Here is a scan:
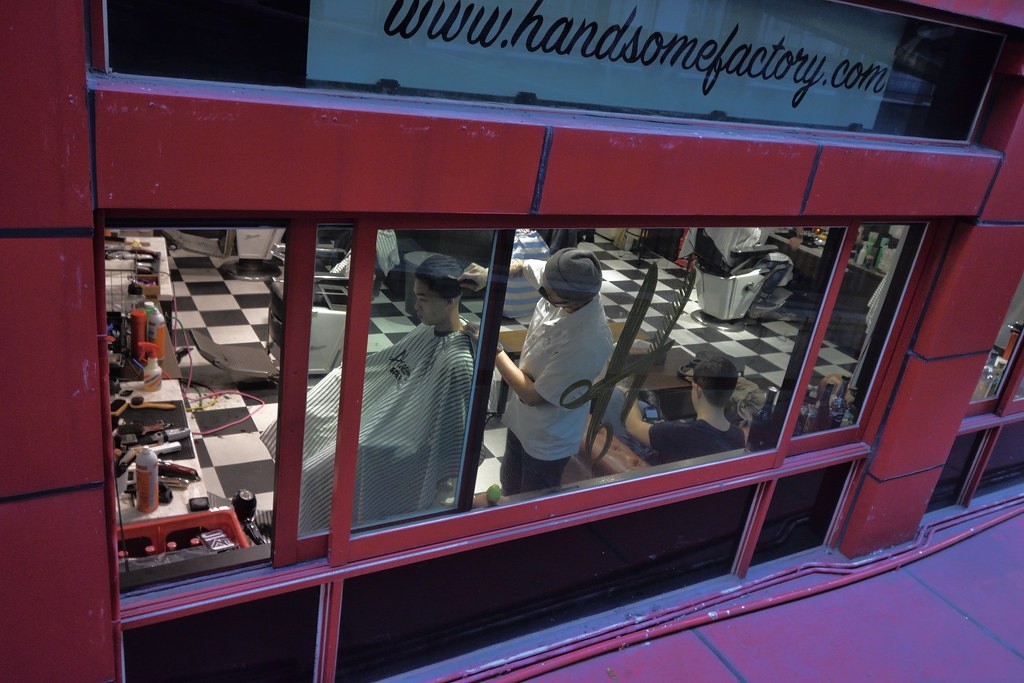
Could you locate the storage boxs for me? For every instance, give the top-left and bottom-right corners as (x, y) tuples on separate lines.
(116, 509), (250, 573)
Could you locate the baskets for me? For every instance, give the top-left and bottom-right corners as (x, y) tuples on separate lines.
(106, 249), (139, 320)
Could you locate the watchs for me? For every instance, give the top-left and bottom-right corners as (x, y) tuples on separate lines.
(497, 340), (504, 352)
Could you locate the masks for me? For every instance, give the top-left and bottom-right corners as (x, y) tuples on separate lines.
(538, 285), (573, 309)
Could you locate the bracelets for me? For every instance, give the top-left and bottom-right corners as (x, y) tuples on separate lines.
(624, 389), (629, 396)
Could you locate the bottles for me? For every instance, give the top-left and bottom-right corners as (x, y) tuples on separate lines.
(868, 231), (887, 269)
(124, 281), (145, 323)
(149, 308), (166, 360)
(855, 243), (868, 266)
(742, 374), (861, 456)
(136, 445), (159, 513)
(141, 302), (156, 317)
(124, 315), (133, 356)
(987, 361), (1007, 398)
(877, 236), (899, 274)
(969, 351), (1000, 403)
(138, 341), (162, 393)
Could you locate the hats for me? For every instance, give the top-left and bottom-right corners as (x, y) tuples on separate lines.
(544, 248), (602, 305)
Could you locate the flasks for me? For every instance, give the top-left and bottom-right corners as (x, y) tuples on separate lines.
(130, 310), (146, 357)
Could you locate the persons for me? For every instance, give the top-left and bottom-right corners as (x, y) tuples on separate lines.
(705, 227), (794, 311)
(590, 354), (747, 465)
(249, 252), (477, 546)
(456, 244), (615, 497)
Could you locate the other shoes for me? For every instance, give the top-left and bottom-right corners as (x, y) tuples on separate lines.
(755, 298), (778, 311)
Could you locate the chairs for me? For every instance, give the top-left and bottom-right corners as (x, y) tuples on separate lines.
(189, 235), (381, 380)
(693, 227), (794, 332)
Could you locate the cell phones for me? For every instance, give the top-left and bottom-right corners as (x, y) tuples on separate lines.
(644, 407), (661, 423)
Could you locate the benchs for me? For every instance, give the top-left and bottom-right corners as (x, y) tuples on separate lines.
(496, 320), (694, 422)
(497, 260), (696, 465)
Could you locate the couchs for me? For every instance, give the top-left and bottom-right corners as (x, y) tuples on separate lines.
(560, 412), (751, 483)
